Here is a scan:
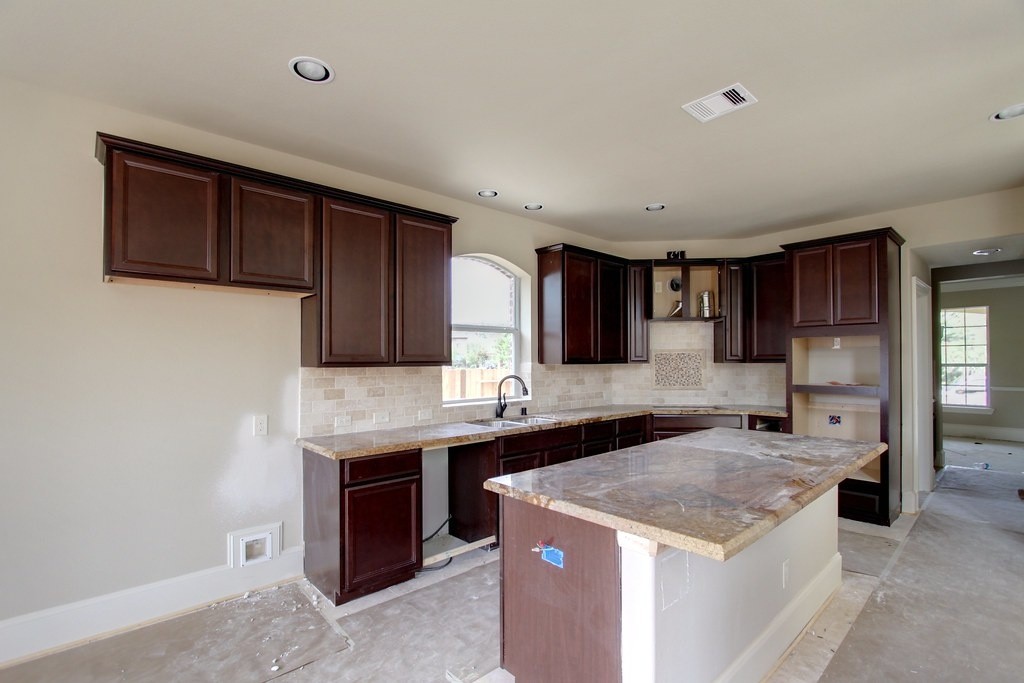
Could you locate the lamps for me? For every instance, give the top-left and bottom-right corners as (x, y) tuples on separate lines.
(644, 203), (666, 211)
(288, 56), (333, 85)
(477, 189), (499, 198)
(989, 103), (1024, 121)
(523, 203), (543, 211)
(973, 248), (1002, 256)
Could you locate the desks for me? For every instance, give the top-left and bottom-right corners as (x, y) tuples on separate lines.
(482, 425), (888, 683)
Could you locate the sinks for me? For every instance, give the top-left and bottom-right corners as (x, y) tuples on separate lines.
(509, 416), (557, 426)
(472, 420), (527, 432)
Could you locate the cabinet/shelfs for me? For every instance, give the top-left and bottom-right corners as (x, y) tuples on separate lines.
(714, 250), (788, 363)
(747, 415), (789, 434)
(101, 150), (315, 299)
(302, 448), (423, 606)
(649, 414), (747, 442)
(647, 260), (725, 321)
(299, 194), (452, 369)
(448, 413), (649, 552)
(535, 242), (649, 364)
(791, 238), (901, 526)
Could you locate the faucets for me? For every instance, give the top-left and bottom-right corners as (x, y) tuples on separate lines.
(495, 375), (528, 418)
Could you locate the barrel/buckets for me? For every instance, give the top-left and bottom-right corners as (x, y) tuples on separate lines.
(697, 290), (715, 318)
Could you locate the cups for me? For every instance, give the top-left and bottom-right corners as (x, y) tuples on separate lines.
(669, 300), (682, 317)
